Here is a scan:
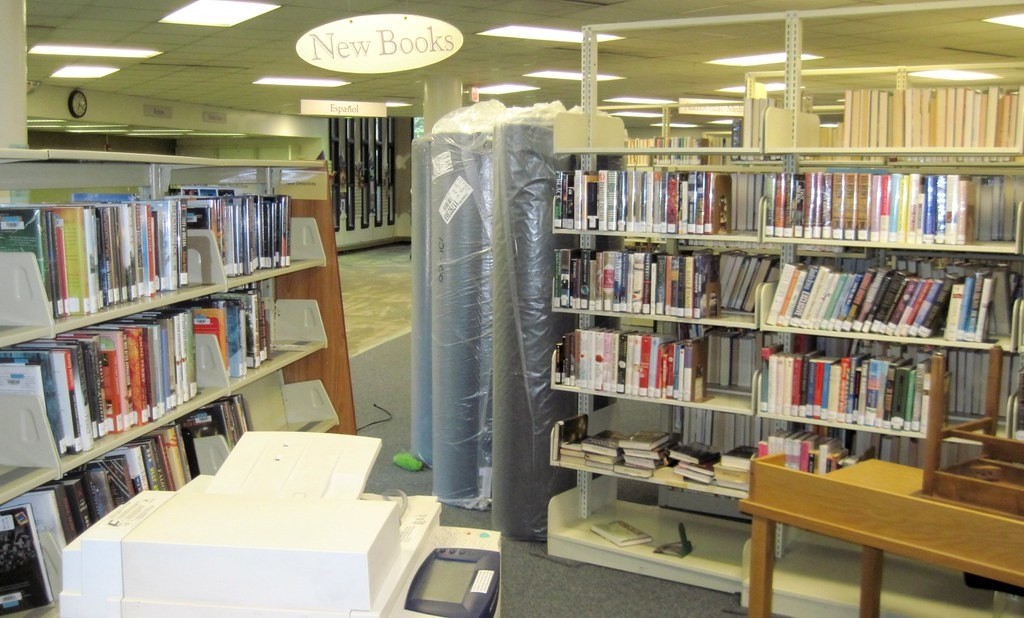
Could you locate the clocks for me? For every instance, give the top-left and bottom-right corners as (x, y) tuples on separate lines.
(68, 90), (87, 118)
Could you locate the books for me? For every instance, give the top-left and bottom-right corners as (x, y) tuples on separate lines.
(0, 194), (292, 615)
(590, 520), (652, 548)
(553, 88), (1024, 492)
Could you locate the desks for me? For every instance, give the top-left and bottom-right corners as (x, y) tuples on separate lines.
(739, 452), (1024, 618)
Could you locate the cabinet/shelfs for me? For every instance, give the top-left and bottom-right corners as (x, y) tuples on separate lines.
(0, 150), (358, 618)
(547, 0), (1024, 618)
